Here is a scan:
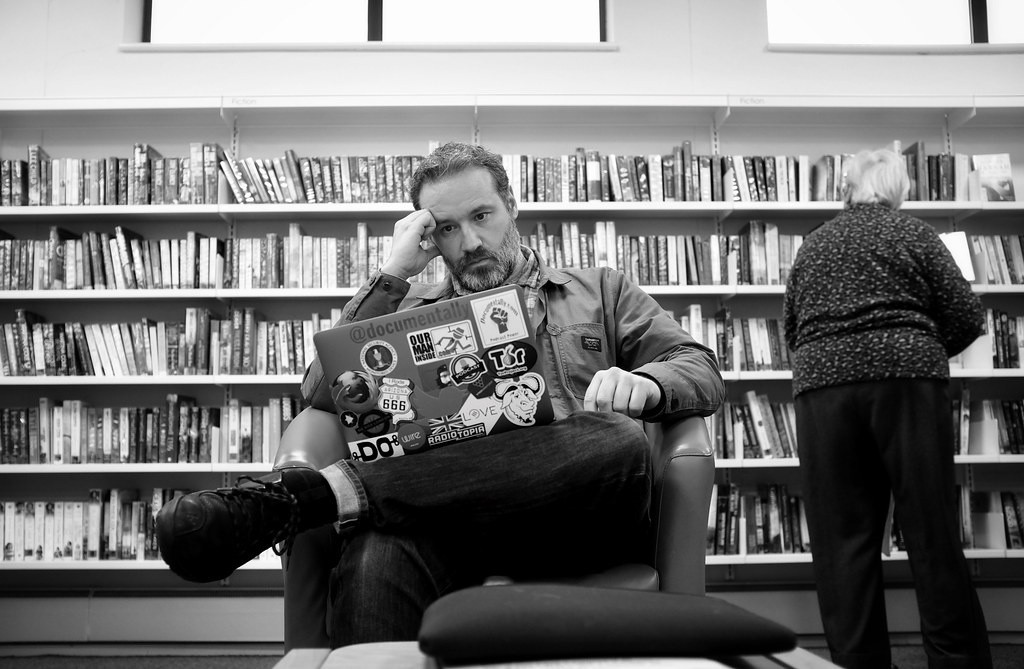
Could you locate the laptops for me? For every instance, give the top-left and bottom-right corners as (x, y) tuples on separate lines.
(313, 284), (556, 460)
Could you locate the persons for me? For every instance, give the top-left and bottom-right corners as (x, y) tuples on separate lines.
(155, 138), (726, 649)
(781, 146), (1003, 669)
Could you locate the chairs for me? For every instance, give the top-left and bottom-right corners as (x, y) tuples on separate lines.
(274, 405), (713, 653)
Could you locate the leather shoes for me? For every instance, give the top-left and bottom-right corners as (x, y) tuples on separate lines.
(156, 467), (339, 583)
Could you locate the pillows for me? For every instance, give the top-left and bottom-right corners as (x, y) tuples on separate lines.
(415, 586), (795, 661)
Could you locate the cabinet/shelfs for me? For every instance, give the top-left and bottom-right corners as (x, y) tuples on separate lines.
(1, 200), (1023, 596)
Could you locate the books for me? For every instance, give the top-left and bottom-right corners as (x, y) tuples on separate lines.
(0, 144), (1024, 566)
